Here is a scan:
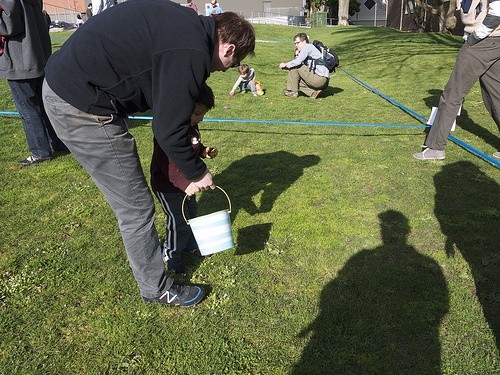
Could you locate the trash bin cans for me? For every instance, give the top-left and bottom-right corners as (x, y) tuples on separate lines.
(311, 13), (316, 28)
(315, 12), (327, 28)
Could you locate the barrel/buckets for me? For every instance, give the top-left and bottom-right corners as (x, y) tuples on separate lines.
(182, 185), (235, 256)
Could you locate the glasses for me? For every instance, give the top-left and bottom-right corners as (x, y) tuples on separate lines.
(231, 49), (240, 68)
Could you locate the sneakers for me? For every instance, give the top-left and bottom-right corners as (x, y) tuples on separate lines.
(141, 283), (205, 308)
(493, 151), (500, 159)
(412, 145), (446, 160)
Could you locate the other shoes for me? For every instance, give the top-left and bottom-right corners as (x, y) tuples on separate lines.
(18, 155), (53, 166)
(309, 89), (322, 98)
(183, 247), (201, 257)
(169, 265), (187, 277)
(284, 89), (299, 97)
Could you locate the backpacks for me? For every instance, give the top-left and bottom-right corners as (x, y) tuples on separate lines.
(306, 39), (339, 74)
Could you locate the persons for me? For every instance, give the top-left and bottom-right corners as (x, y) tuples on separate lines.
(0, 0), (69, 166)
(279, 33), (330, 99)
(149, 82), (218, 271)
(230, 63), (265, 98)
(76, 0), (120, 29)
(41, 10), (51, 32)
(42, 0), (255, 309)
(178, 0), (224, 16)
(412, 0), (500, 161)
